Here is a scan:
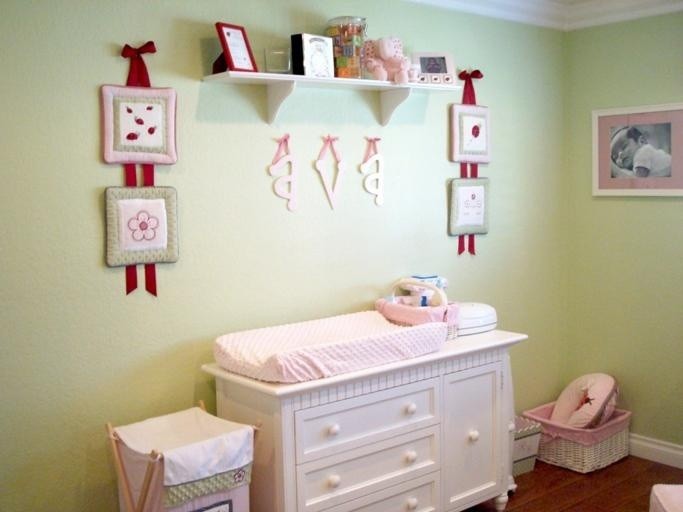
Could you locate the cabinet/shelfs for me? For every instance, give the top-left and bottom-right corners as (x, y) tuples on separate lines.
(199, 333), (527, 511)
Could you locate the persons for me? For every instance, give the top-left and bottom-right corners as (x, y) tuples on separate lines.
(610, 127), (671, 177)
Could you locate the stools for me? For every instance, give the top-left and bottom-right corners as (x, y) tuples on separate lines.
(647, 484), (682, 512)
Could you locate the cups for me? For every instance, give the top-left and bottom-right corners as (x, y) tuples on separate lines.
(264, 47), (291, 73)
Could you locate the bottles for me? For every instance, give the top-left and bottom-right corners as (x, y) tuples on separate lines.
(325, 15), (368, 78)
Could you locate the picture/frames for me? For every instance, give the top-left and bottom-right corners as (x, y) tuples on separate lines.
(211, 18), (257, 71)
(591, 101), (683, 196)
(412, 50), (457, 85)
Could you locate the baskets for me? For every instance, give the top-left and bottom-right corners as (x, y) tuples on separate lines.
(523, 401), (631, 474)
(377, 280), (447, 326)
